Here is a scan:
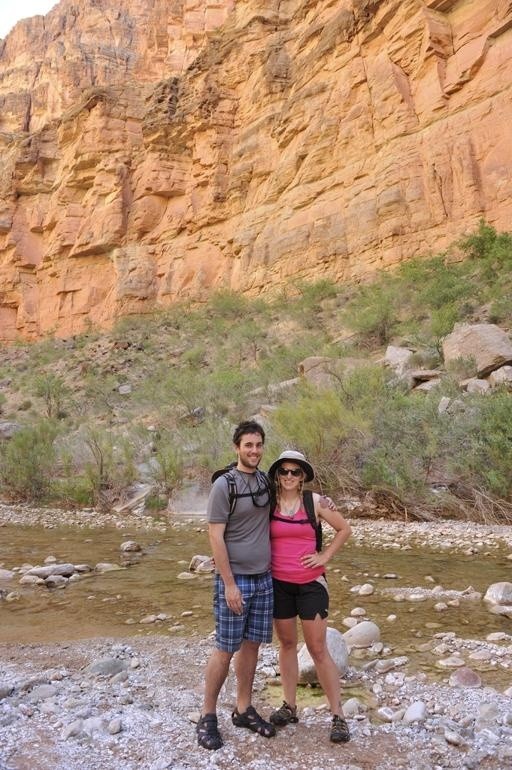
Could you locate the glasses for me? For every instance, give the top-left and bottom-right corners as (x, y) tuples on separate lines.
(276, 467), (303, 477)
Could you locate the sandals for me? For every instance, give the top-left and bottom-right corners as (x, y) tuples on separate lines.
(269, 700), (299, 726)
(231, 705), (276, 738)
(195, 712), (224, 750)
(330, 714), (351, 743)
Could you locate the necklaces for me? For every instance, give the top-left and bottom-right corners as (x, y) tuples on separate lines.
(281, 496), (299, 518)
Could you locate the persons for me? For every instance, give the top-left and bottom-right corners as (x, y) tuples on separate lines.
(195, 420), (337, 749)
(210, 450), (351, 741)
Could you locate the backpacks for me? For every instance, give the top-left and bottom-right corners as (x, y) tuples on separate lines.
(270, 490), (322, 555)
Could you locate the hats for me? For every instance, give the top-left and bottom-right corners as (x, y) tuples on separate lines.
(211, 461), (239, 485)
(267, 449), (315, 484)
